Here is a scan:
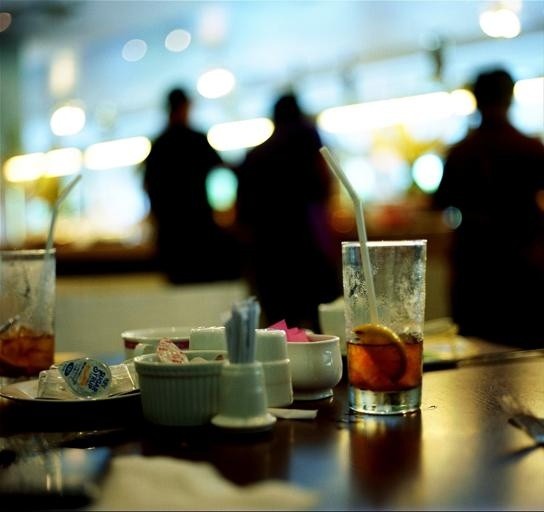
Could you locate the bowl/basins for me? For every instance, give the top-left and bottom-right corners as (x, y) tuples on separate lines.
(134, 350), (229, 427)
(120, 326), (198, 360)
(189, 328), (294, 408)
(286, 334), (344, 401)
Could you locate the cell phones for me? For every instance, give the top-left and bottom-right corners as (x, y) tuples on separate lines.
(0, 445), (111, 506)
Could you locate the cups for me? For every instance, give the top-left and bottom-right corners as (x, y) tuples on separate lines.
(1, 247), (57, 379)
(341, 239), (428, 416)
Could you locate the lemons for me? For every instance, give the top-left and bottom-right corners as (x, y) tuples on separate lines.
(353, 323), (408, 383)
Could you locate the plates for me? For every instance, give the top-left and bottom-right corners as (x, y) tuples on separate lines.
(1, 362), (142, 401)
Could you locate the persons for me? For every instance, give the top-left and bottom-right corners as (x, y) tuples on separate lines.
(427, 68), (543, 352)
